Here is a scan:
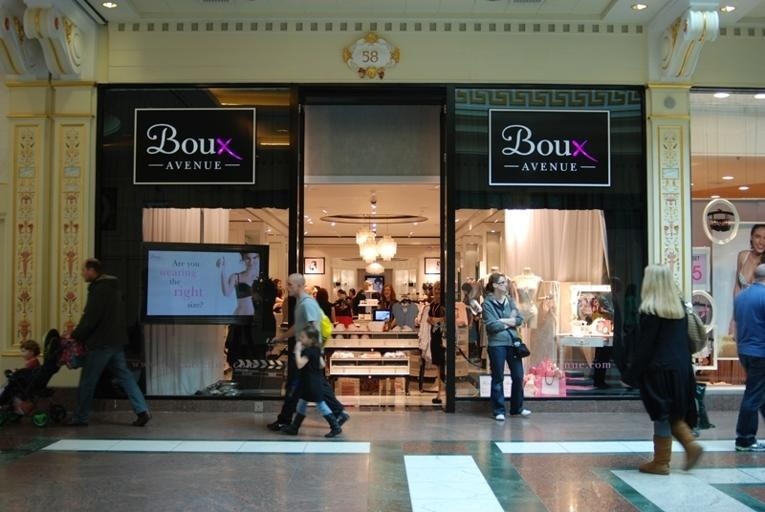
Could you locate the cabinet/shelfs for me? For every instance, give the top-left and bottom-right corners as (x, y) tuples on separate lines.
(324, 285), (426, 395)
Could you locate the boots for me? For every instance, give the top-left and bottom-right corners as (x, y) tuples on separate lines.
(672, 421), (702, 470)
(638, 437), (672, 475)
(323, 414), (342, 437)
(278, 413), (306, 435)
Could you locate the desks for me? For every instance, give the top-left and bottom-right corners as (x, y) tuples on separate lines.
(556, 332), (613, 371)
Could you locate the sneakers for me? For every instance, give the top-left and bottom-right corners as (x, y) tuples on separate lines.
(132, 409), (151, 426)
(521, 409), (532, 417)
(736, 440), (765, 452)
(495, 413), (505, 420)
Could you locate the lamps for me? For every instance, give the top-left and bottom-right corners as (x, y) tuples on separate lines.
(355, 216), (397, 274)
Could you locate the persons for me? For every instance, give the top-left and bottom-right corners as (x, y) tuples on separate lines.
(215, 252), (259, 316)
(64, 258), (152, 428)
(272, 273), (482, 438)
(732, 263), (765, 452)
(482, 272), (532, 420)
(728, 224), (765, 337)
(19, 340), (41, 368)
(638, 263), (704, 476)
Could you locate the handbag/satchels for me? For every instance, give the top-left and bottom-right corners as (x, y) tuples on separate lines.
(535, 376), (567, 398)
(320, 314), (334, 349)
(686, 301), (706, 354)
(511, 337), (530, 359)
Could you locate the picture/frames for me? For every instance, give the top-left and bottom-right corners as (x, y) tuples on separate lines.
(691, 326), (717, 371)
(712, 221), (765, 361)
(424, 257), (440, 274)
(692, 247), (711, 293)
(303, 256), (325, 275)
(139, 241), (270, 324)
(693, 290), (716, 334)
(570, 285), (614, 330)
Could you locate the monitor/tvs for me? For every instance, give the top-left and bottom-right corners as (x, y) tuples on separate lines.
(374, 309), (391, 320)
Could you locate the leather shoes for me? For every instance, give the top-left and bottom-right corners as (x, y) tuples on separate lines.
(336, 412), (349, 427)
(267, 420), (291, 431)
